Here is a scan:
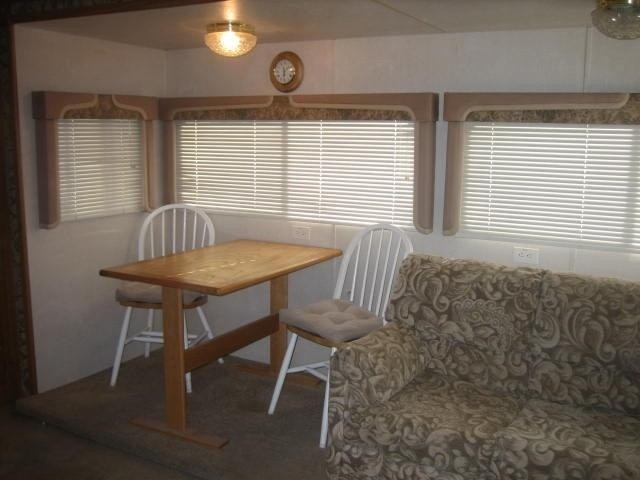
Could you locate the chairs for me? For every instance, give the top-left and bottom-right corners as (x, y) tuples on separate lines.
(109, 203), (226, 395)
(266, 222), (416, 451)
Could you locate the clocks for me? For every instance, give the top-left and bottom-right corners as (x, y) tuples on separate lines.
(268, 51), (304, 92)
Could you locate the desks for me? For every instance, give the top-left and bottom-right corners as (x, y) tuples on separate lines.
(100, 240), (345, 451)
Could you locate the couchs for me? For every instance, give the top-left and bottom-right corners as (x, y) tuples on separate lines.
(320, 252), (639, 478)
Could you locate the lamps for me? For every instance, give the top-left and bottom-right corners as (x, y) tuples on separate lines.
(590, 1), (639, 41)
(203, 22), (257, 59)
